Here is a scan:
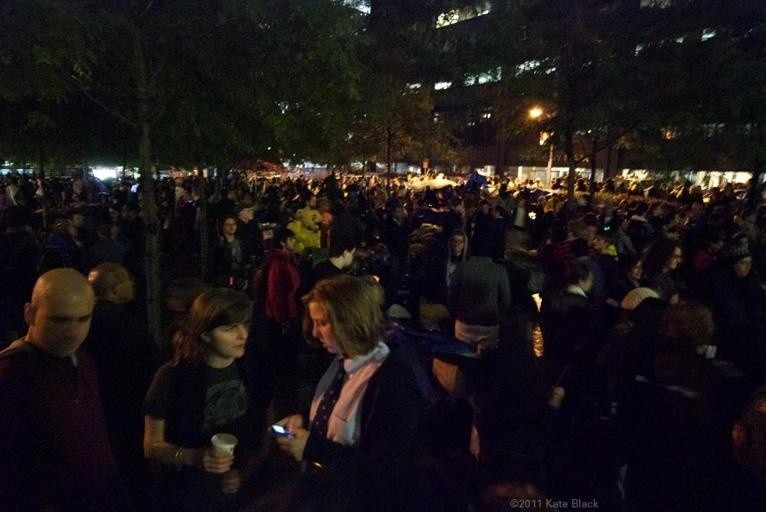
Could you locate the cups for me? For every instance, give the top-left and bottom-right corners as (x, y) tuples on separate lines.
(211, 434), (237, 456)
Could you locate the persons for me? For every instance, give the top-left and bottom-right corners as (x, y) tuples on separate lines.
(0, 162), (766, 512)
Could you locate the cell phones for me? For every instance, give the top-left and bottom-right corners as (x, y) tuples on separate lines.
(271, 424), (293, 440)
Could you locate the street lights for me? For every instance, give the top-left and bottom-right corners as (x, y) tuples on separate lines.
(528, 106), (554, 184)
